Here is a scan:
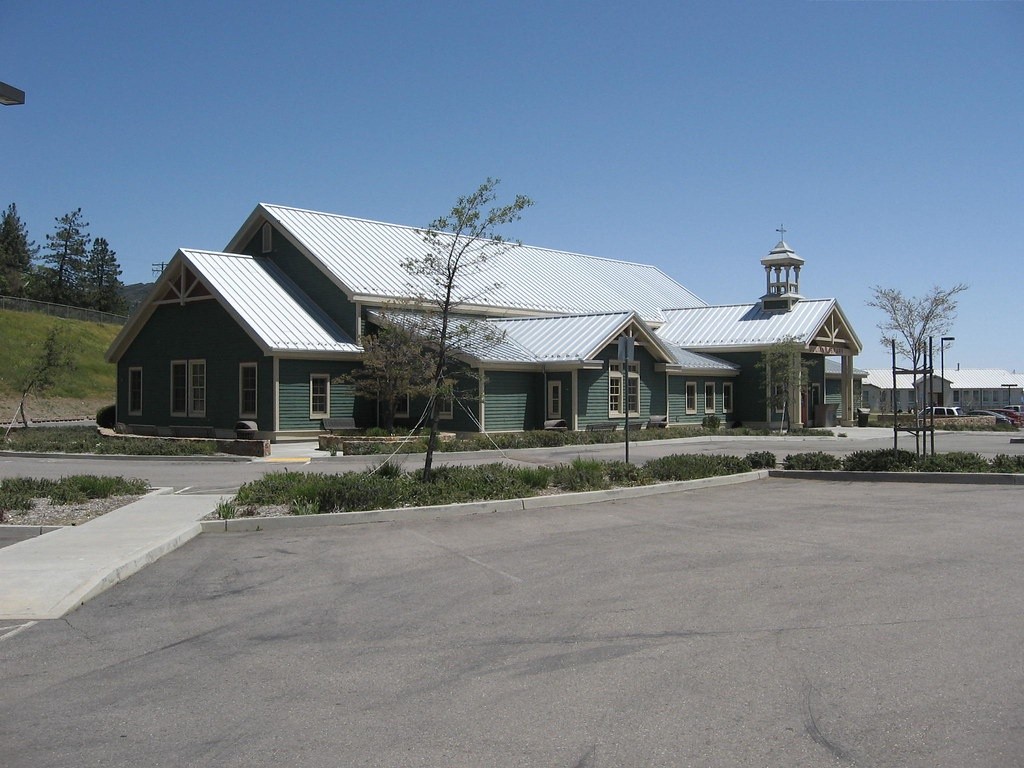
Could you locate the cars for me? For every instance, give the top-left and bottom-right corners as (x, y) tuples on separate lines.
(965, 405), (1024, 429)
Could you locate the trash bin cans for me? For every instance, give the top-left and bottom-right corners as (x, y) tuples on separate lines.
(650, 416), (667, 428)
(233, 421), (259, 440)
(544, 419), (567, 432)
(857, 408), (870, 427)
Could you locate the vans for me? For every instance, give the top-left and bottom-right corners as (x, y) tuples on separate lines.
(918, 406), (965, 417)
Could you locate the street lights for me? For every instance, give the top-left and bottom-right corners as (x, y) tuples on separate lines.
(1001, 383), (1018, 405)
(941, 335), (956, 406)
